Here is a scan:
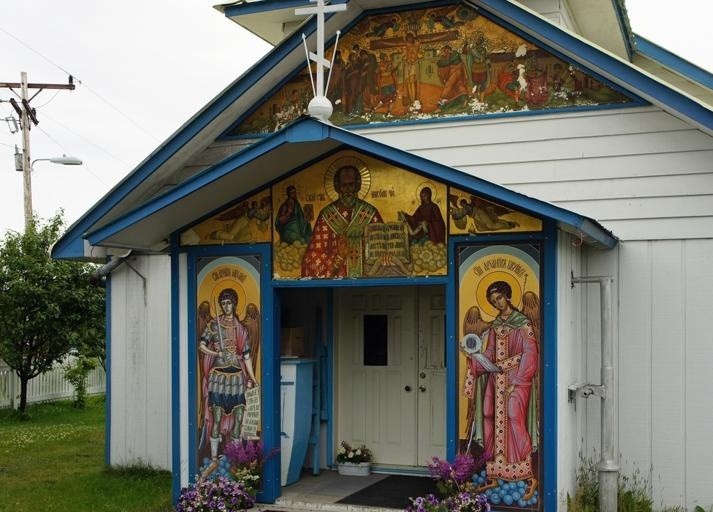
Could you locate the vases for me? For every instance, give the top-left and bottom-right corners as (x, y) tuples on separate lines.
(337, 462), (370, 475)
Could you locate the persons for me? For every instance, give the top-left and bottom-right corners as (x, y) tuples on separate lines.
(194, 288), (257, 465)
(299, 165), (386, 280)
(397, 186), (445, 248)
(447, 199), (520, 231)
(287, 90), (305, 115)
(454, 278), (538, 501)
(203, 201), (271, 242)
(272, 185), (313, 244)
(320, 13), (568, 112)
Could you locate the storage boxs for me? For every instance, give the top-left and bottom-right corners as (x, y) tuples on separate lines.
(280, 326), (305, 357)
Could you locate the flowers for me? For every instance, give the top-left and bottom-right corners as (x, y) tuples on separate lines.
(335, 439), (373, 463)
(404, 452), (494, 512)
(177, 437), (279, 512)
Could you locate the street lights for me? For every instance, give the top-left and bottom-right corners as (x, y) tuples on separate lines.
(21, 72), (82, 235)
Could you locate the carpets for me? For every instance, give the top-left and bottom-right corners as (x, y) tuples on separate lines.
(334, 475), (448, 508)
